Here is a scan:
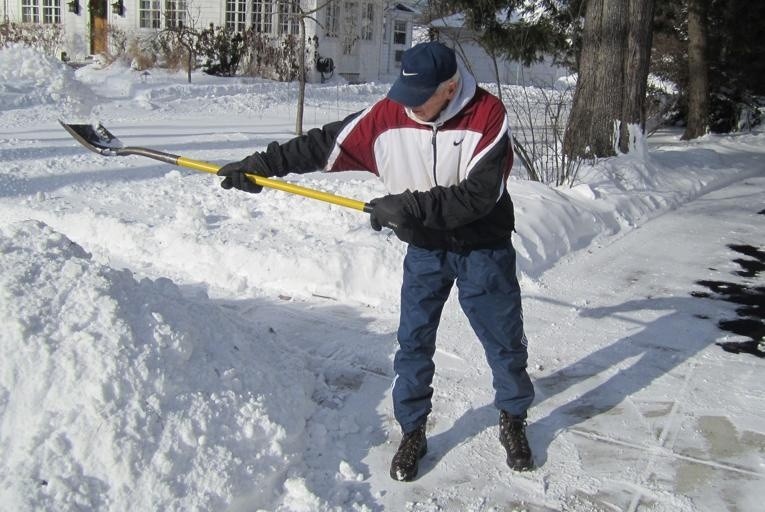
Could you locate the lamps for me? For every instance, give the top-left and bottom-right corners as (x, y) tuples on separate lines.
(111, 0), (123, 15)
(65, 0), (80, 14)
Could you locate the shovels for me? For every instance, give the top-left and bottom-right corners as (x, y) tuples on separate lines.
(57, 118), (377, 215)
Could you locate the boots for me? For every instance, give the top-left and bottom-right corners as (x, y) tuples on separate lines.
(388, 411), (428, 484)
(499, 408), (533, 472)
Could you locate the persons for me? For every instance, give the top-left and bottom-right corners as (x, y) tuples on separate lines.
(216, 41), (536, 482)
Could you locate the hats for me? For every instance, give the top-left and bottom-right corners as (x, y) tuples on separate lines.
(386, 41), (459, 107)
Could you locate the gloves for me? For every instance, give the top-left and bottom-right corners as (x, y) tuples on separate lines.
(216, 151), (272, 194)
(366, 188), (423, 233)
